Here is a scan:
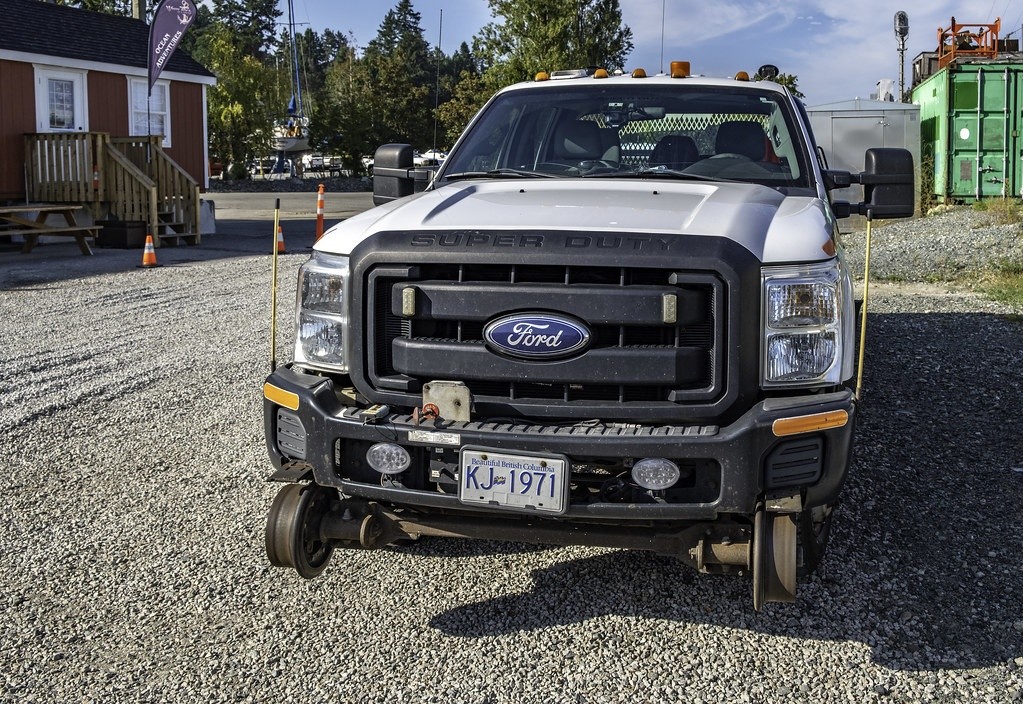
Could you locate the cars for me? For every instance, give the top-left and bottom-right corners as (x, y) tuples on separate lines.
(225, 150), (374, 181)
(412, 148), (448, 168)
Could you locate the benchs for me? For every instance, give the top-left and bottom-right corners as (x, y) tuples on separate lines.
(0, 225), (104, 237)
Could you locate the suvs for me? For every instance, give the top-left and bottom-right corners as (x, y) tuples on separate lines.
(260, 59), (915, 612)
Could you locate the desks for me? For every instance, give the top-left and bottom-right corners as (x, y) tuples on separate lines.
(0, 204), (93, 256)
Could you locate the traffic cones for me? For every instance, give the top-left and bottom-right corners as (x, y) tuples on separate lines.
(136, 236), (164, 268)
(269, 226), (288, 255)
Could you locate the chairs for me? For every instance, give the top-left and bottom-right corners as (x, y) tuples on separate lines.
(596, 127), (621, 164)
(648, 135), (698, 171)
(556, 120), (601, 168)
(713, 120), (769, 164)
